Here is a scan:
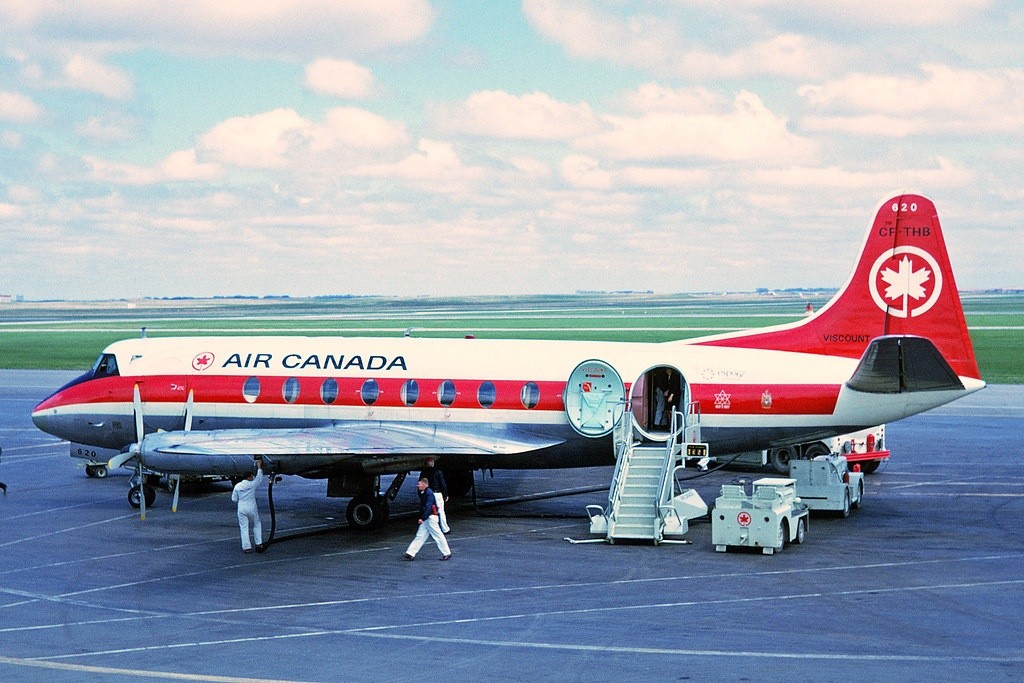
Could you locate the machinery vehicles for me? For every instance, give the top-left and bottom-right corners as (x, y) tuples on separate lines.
(711, 478), (809, 554)
(789, 451), (865, 518)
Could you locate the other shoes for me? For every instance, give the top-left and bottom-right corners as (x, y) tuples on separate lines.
(441, 553), (453, 560)
(255, 545), (264, 553)
(243, 548), (253, 554)
(403, 552), (414, 560)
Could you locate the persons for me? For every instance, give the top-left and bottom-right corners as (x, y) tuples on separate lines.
(663, 368), (680, 426)
(654, 369), (666, 427)
(418, 458), (452, 535)
(402, 478), (452, 561)
(0, 447), (7, 494)
(232, 461), (265, 553)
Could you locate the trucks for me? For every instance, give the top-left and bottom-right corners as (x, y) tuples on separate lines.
(704, 425), (890, 475)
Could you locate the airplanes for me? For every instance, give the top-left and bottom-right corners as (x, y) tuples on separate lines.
(31, 195), (987, 531)
